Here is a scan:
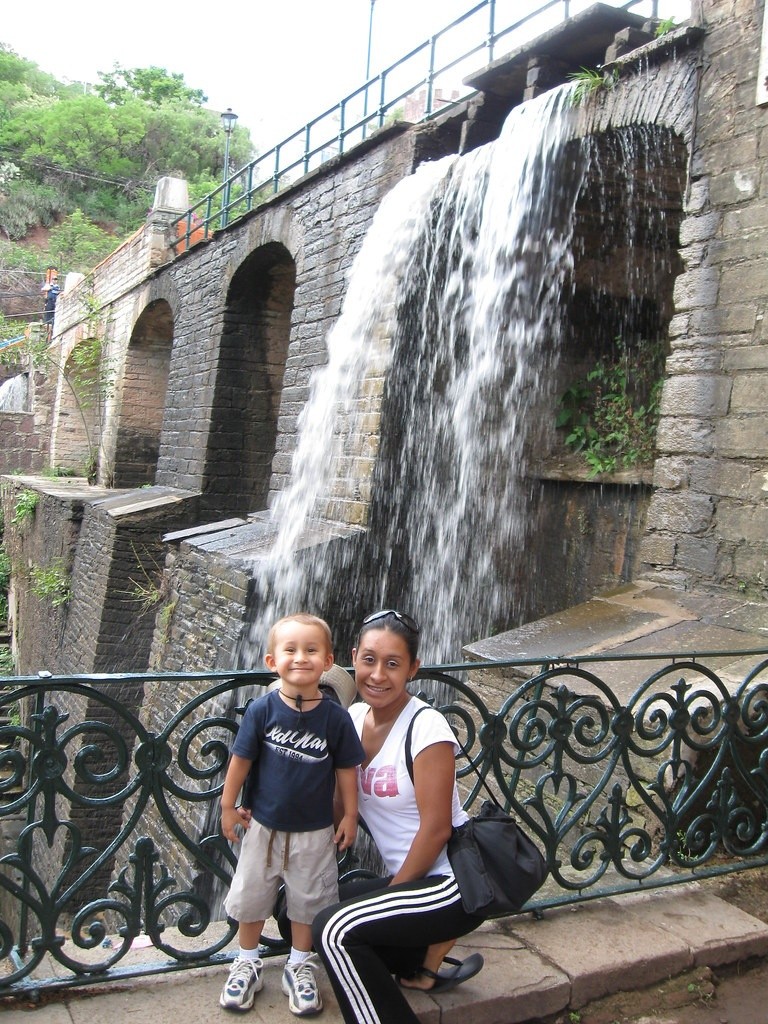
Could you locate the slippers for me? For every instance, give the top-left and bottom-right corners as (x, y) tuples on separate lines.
(395, 952), (484, 994)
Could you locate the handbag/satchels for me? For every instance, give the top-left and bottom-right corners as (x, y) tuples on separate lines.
(404, 706), (549, 915)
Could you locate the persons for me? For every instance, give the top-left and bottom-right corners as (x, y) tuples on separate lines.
(219, 614), (367, 1016)
(237, 610), (484, 1024)
(45, 276), (61, 324)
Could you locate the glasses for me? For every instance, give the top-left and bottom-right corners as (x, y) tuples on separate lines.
(362, 609), (420, 636)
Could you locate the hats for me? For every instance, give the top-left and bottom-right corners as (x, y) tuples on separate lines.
(264, 664), (358, 711)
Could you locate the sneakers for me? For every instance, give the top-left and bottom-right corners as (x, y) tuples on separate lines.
(219, 957), (264, 1010)
(282, 952), (324, 1015)
